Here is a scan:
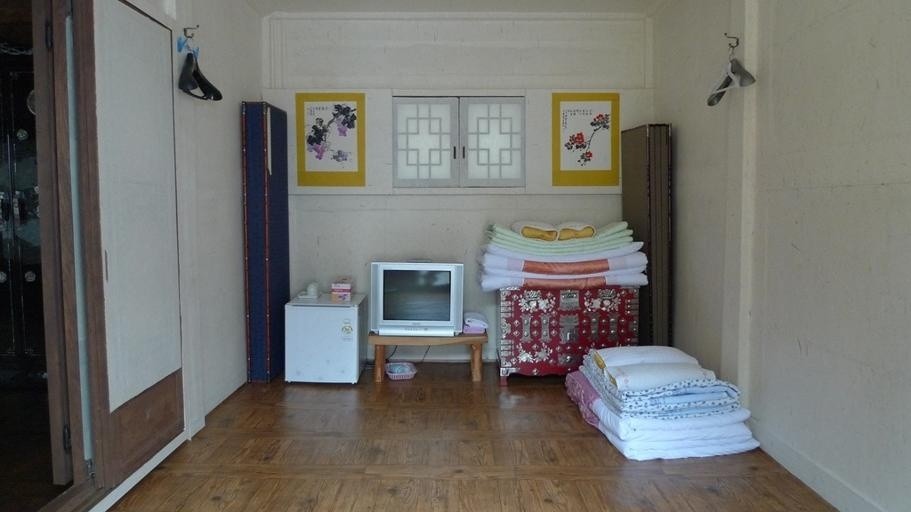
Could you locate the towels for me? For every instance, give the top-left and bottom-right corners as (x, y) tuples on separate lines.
(464, 311), (487, 333)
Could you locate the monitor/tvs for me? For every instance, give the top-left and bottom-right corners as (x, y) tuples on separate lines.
(368, 262), (463, 337)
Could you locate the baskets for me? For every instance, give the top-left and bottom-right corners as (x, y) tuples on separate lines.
(385, 362), (416, 380)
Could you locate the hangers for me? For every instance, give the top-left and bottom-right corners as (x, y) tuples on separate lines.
(704, 42), (758, 108)
(179, 35), (223, 102)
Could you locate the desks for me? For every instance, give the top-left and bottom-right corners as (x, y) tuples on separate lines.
(368, 329), (487, 383)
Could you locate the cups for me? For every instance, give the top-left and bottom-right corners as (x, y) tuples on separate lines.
(307, 281), (321, 296)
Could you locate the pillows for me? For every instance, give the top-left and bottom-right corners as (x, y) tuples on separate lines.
(512, 221), (599, 241)
(594, 344), (717, 392)
